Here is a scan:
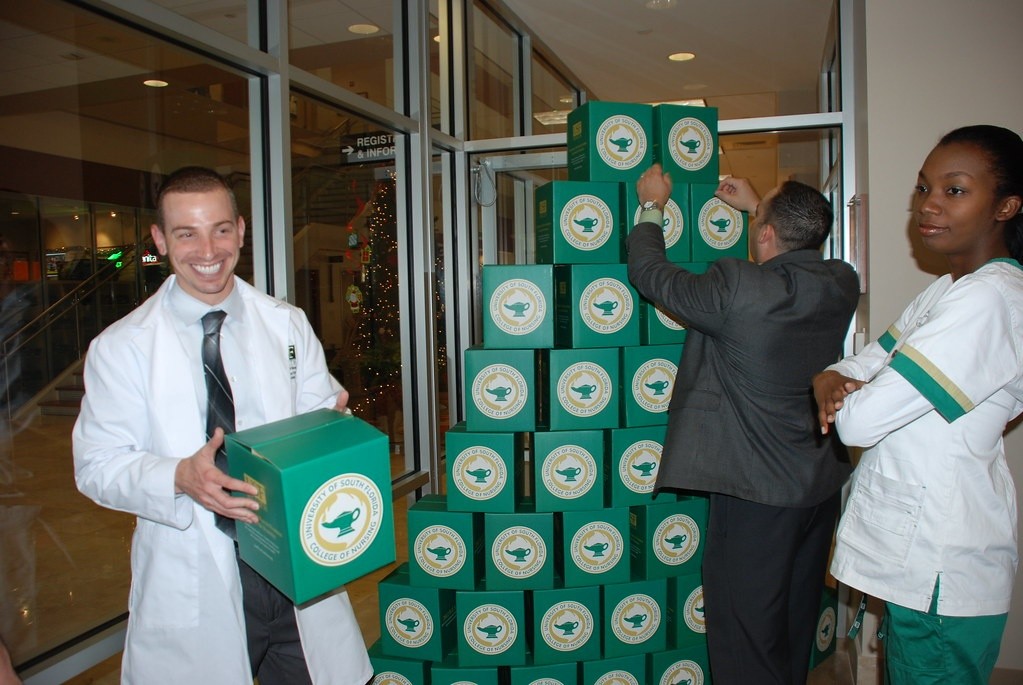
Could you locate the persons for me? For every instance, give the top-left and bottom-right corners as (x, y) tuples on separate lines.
(811, 125), (1023, 685)
(69, 165), (379, 685)
(626, 164), (859, 685)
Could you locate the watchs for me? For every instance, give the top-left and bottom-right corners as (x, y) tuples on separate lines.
(641, 200), (664, 215)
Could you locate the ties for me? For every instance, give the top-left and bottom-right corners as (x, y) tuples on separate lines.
(199, 310), (238, 542)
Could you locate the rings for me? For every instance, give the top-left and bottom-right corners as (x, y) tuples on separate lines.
(640, 176), (644, 178)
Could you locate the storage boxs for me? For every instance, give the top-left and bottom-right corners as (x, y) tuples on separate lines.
(224, 100), (837, 685)
